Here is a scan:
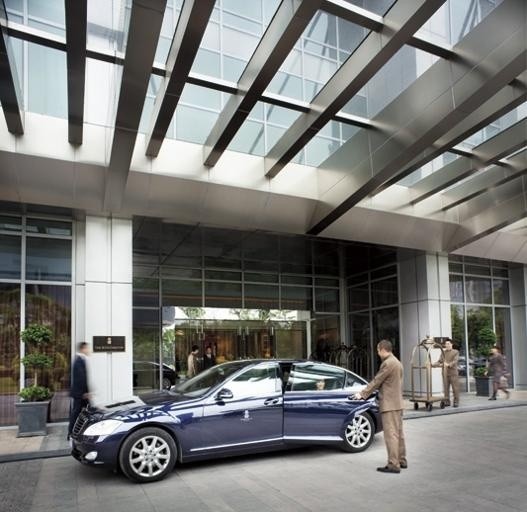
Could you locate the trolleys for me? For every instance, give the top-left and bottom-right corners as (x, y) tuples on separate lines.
(328, 343), (369, 383)
(407, 336), (450, 412)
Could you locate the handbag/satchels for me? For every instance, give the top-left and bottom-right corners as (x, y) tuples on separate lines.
(499, 375), (509, 388)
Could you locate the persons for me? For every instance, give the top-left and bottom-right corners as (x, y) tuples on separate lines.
(283, 369), (291, 390)
(204, 346), (216, 371)
(316, 379), (325, 389)
(434, 339), (461, 408)
(352, 340), (407, 473)
(315, 331), (330, 360)
(187, 345), (199, 379)
(65, 341), (93, 442)
(484, 346), (510, 400)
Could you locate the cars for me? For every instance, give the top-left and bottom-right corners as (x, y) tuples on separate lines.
(456, 357), (475, 375)
(132, 361), (178, 392)
(68, 356), (384, 484)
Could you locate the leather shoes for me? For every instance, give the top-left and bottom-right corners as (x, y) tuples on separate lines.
(377, 465), (401, 473)
(443, 391), (511, 407)
(399, 463), (408, 468)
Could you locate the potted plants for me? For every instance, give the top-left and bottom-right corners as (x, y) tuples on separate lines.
(14, 324), (57, 436)
(473, 326), (497, 398)
